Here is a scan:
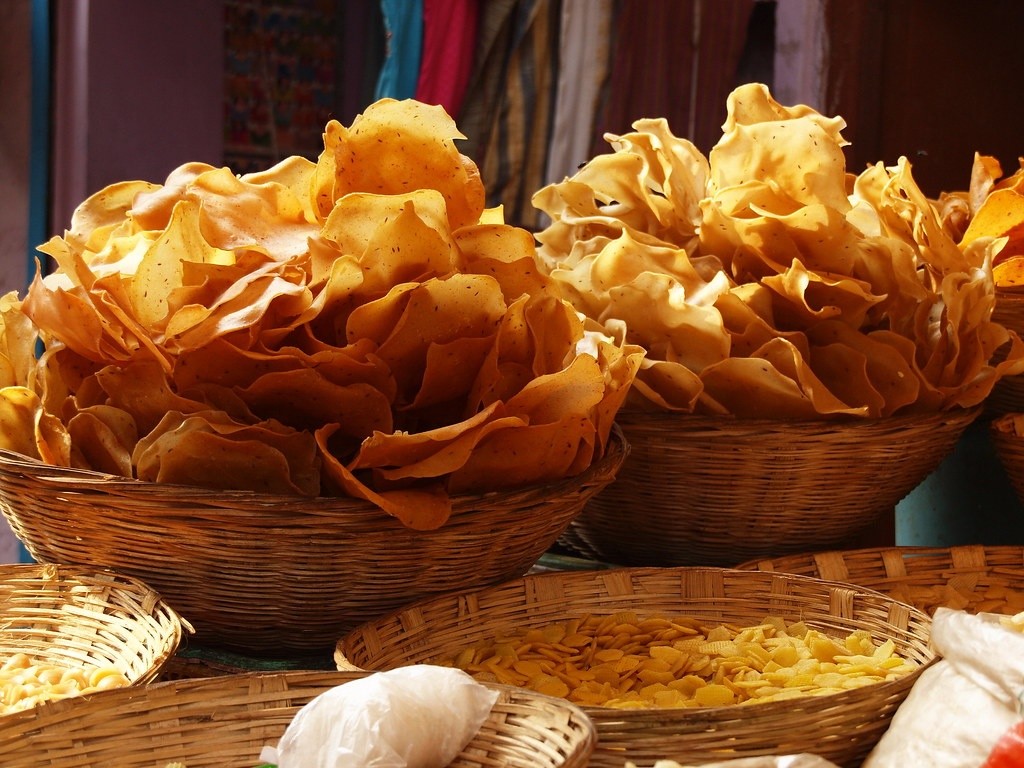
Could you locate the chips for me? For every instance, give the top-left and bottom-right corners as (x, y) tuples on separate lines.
(1, 83), (1024, 533)
(434, 613), (917, 710)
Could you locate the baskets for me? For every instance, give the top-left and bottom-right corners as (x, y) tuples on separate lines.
(1, 408), (1024, 768)
